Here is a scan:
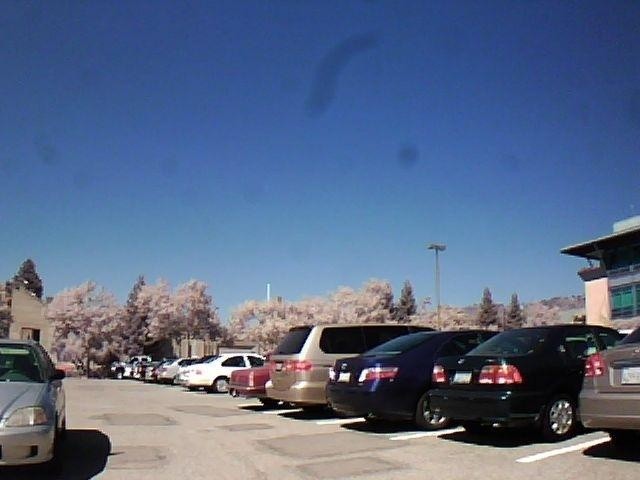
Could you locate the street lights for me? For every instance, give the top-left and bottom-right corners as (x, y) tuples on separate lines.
(428, 243), (445, 330)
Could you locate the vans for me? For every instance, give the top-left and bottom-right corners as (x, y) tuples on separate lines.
(262, 320), (439, 416)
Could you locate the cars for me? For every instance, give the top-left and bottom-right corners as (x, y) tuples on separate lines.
(111, 352), (269, 399)
(579, 324), (640, 451)
(326, 327), (501, 429)
(0, 339), (67, 471)
(428, 323), (627, 437)
